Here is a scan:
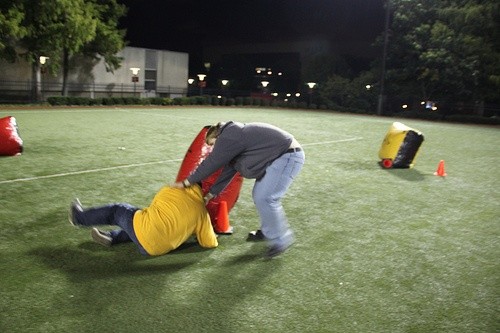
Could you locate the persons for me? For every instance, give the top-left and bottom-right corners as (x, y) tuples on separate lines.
(70, 179), (219, 257)
(183, 121), (305, 256)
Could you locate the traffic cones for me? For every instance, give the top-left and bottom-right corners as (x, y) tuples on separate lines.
(433, 160), (448, 177)
(213, 200), (234, 235)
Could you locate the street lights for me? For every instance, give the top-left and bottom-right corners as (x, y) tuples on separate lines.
(221, 79), (229, 97)
(261, 81), (270, 96)
(130, 67), (141, 97)
(197, 73), (207, 96)
(306, 82), (317, 108)
(188, 78), (195, 97)
(39, 55), (49, 102)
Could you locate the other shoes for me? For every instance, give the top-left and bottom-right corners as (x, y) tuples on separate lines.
(91, 228), (113, 246)
(249, 229), (265, 240)
(69, 198), (84, 228)
(268, 237), (295, 257)
(214, 227), (233, 235)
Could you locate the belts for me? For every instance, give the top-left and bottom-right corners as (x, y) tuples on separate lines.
(285, 148), (301, 153)
